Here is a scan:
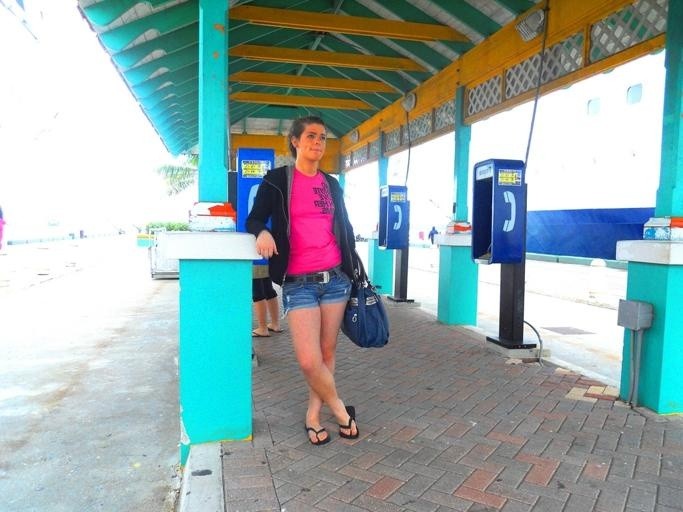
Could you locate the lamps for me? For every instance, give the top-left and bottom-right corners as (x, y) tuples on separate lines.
(514, 9), (546, 41)
(400, 92), (416, 113)
(349, 130), (359, 144)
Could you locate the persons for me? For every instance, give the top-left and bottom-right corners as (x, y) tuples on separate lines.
(252, 279), (284, 338)
(429, 226), (438, 244)
(246, 116), (359, 446)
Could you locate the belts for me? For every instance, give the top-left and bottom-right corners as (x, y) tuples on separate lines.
(285, 268), (343, 284)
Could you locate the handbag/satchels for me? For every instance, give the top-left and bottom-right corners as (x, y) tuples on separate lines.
(341, 280), (389, 348)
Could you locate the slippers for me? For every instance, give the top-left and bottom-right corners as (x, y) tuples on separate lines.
(305, 424), (330, 445)
(252, 331), (270, 337)
(340, 405), (359, 439)
(267, 327), (283, 333)
